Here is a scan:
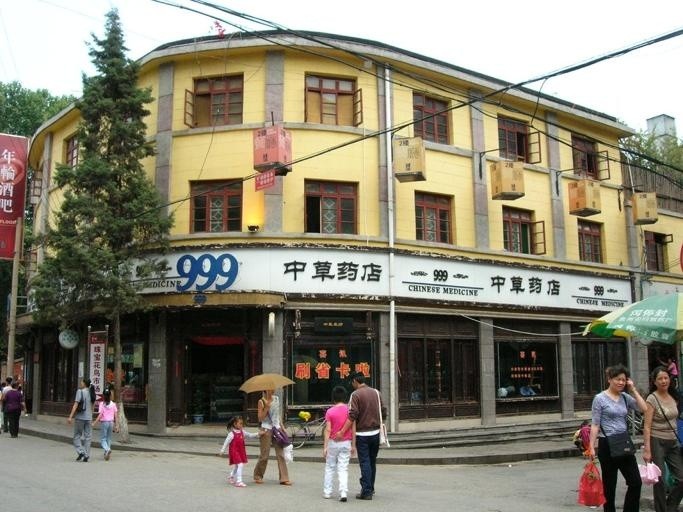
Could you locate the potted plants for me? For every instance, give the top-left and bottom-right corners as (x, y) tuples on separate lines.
(190, 386), (204, 425)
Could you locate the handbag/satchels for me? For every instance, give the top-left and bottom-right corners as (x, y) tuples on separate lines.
(379, 424), (390, 448)
(75, 397), (86, 412)
(607, 431), (636, 457)
(272, 426), (290, 448)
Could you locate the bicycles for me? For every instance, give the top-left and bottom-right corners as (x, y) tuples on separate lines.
(284, 403), (329, 451)
(626, 407), (644, 437)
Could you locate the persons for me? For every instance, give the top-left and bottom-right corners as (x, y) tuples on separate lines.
(585, 363), (648, 512)
(67, 377), (95, 461)
(218, 415), (266, 488)
(642, 365), (682, 512)
(0, 376), (28, 441)
(335, 369), (387, 500)
(92, 388), (118, 461)
(252, 391), (293, 485)
(656, 357), (678, 388)
(321, 386), (357, 501)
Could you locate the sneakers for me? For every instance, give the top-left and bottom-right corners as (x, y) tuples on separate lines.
(356, 494), (373, 500)
(281, 481), (292, 485)
(338, 497), (347, 501)
(104, 448), (112, 460)
(76, 452), (89, 462)
(255, 479), (263, 483)
(227, 476), (247, 487)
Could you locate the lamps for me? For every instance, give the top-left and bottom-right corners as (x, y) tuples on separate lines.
(246, 222), (261, 234)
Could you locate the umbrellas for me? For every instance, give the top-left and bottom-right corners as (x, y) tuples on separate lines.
(580, 289), (683, 404)
(237, 372), (295, 400)
(636, 459), (662, 487)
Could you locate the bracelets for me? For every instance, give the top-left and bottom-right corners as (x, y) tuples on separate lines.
(628, 387), (636, 393)
